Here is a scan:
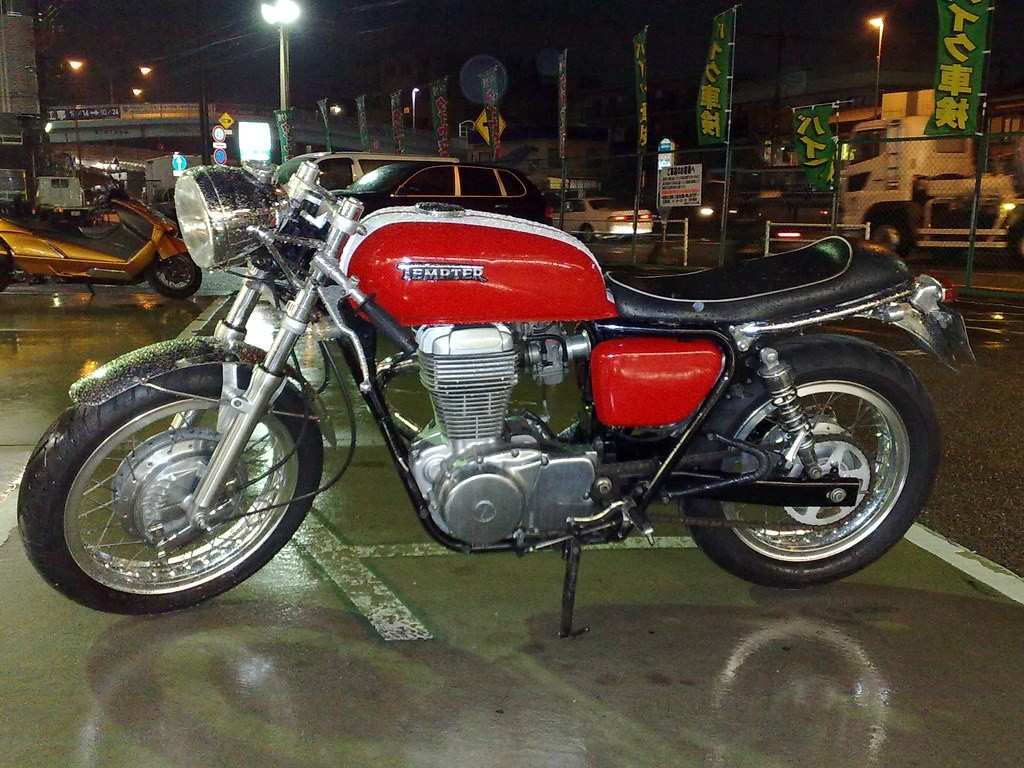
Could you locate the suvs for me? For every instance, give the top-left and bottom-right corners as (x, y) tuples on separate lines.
(277, 145), (458, 193)
(326, 158), (555, 230)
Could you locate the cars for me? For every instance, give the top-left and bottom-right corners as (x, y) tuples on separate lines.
(549, 195), (656, 244)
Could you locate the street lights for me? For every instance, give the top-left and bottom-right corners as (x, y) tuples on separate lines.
(869, 15), (887, 156)
(260, 0), (304, 162)
(140, 63), (163, 119)
(64, 56), (88, 159)
(412, 86), (422, 155)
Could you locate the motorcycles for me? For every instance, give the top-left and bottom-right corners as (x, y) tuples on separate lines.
(0, 160), (204, 300)
(14, 158), (977, 641)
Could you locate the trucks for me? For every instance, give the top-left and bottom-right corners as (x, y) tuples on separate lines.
(838, 113), (1024, 276)
